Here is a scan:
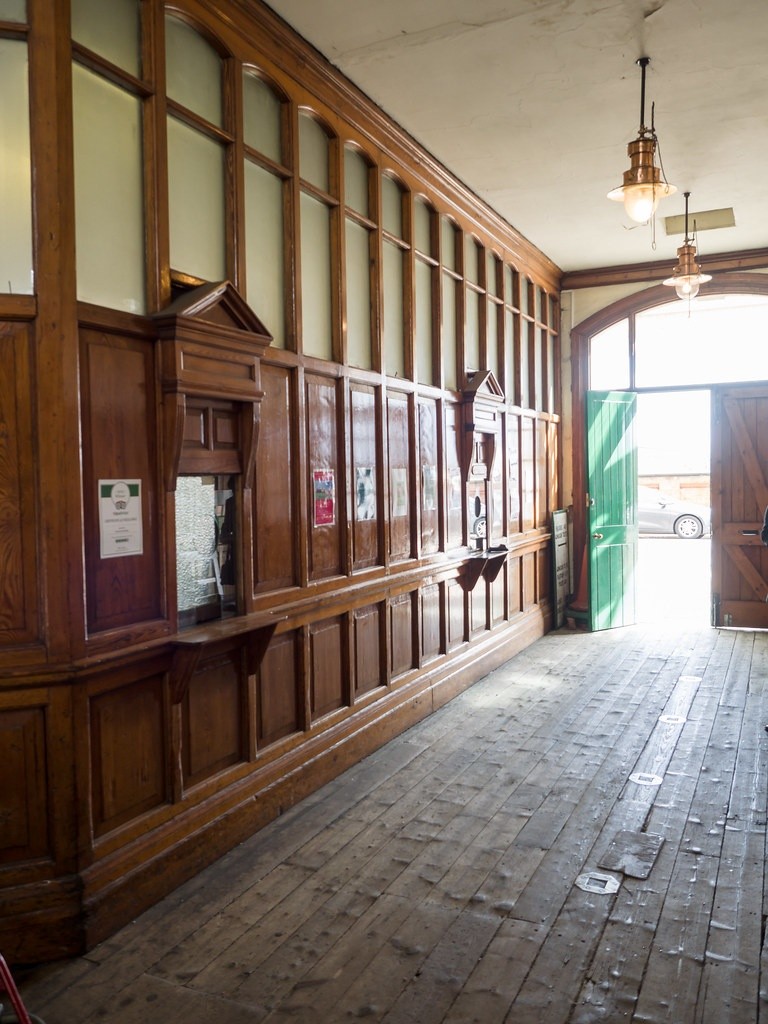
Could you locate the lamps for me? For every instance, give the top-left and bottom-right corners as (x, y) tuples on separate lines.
(608, 52), (678, 224)
(662, 192), (713, 302)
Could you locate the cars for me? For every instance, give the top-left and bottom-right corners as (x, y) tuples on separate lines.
(636, 485), (711, 540)
